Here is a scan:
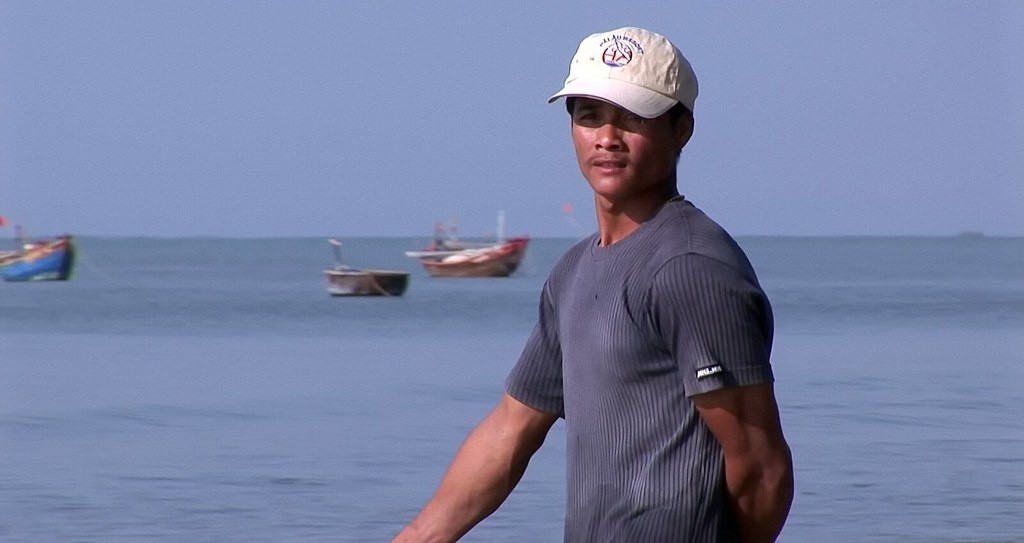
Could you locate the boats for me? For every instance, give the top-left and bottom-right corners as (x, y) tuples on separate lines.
(403, 209), (531, 278)
(320, 238), (412, 298)
(0, 215), (75, 282)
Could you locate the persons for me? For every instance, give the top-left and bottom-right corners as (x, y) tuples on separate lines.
(388, 28), (796, 543)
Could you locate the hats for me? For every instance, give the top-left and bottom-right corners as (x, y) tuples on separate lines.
(547, 26), (699, 120)
